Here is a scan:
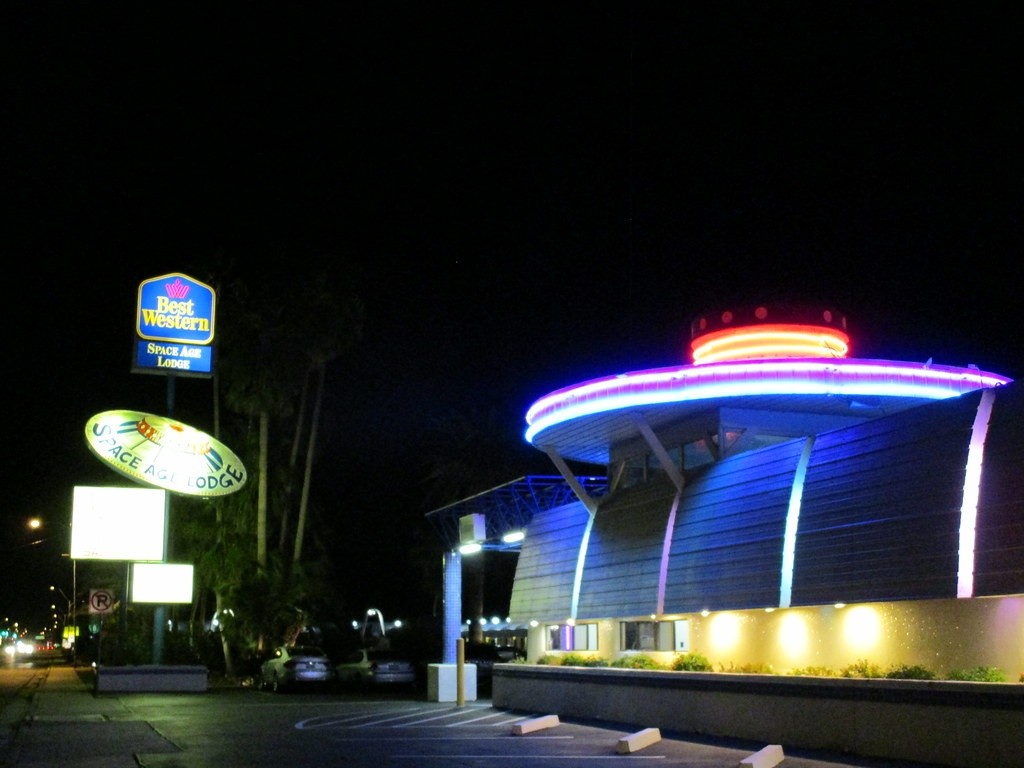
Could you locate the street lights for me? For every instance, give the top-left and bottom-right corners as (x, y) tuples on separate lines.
(49, 586), (71, 633)
(29, 518), (78, 667)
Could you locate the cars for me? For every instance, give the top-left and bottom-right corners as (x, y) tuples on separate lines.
(464, 644), (525, 684)
(255, 644), (416, 696)
